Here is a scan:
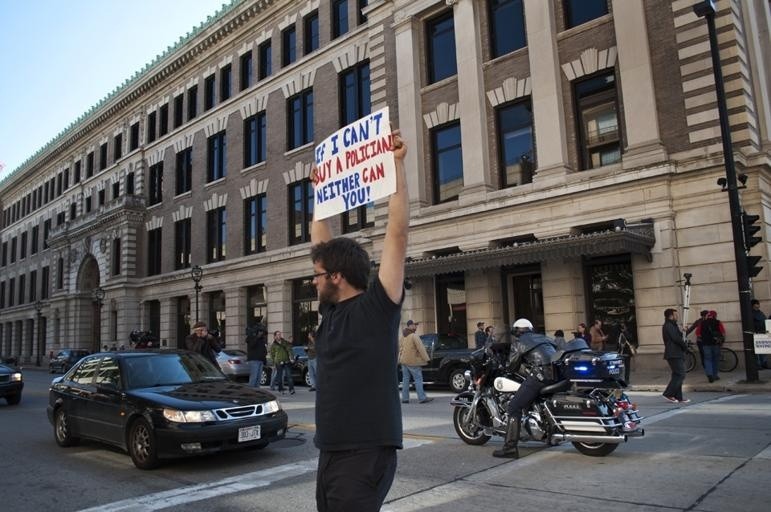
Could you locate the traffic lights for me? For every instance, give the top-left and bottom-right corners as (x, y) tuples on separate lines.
(744, 212), (762, 250)
(743, 255), (764, 279)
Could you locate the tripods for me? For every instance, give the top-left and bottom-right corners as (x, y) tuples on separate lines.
(682, 282), (690, 342)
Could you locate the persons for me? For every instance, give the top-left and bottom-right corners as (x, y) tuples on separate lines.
(751, 298), (770, 370)
(475, 322), (495, 349)
(102, 344), (109, 352)
(685, 310), (709, 370)
(120, 344), (125, 350)
(184, 322), (231, 381)
(271, 336), (294, 391)
(134, 341), (146, 349)
(658, 307), (693, 404)
(305, 323), (317, 392)
(271, 331), (295, 394)
(393, 321), (436, 405)
(160, 340), (168, 350)
(552, 320), (610, 351)
(129, 343), (134, 348)
(307, 120), (414, 511)
(616, 323), (637, 388)
(245, 322), (268, 387)
(110, 343), (117, 351)
(489, 316), (560, 462)
(696, 309), (726, 382)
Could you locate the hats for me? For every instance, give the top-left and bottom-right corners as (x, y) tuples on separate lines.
(407, 319), (419, 326)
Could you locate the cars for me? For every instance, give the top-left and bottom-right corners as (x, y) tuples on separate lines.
(256, 345), (315, 388)
(0, 364), (23, 406)
(48, 351), (289, 470)
(215, 348), (253, 378)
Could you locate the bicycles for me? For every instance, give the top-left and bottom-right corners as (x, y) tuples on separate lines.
(672, 328), (740, 373)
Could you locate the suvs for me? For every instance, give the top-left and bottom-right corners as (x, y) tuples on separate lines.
(47, 350), (90, 375)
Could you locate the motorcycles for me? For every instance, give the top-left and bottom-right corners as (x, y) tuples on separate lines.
(452, 324), (645, 457)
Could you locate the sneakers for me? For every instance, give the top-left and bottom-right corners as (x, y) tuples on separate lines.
(663, 395), (690, 403)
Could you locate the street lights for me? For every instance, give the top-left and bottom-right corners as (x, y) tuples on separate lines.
(690, 1), (767, 383)
(34, 300), (44, 367)
(94, 288), (106, 353)
(190, 265), (203, 325)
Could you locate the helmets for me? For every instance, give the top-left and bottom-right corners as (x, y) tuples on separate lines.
(513, 318), (535, 336)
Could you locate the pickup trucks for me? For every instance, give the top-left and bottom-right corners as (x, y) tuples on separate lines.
(395, 331), (480, 394)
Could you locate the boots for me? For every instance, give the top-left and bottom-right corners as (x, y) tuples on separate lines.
(493, 417), (520, 459)
